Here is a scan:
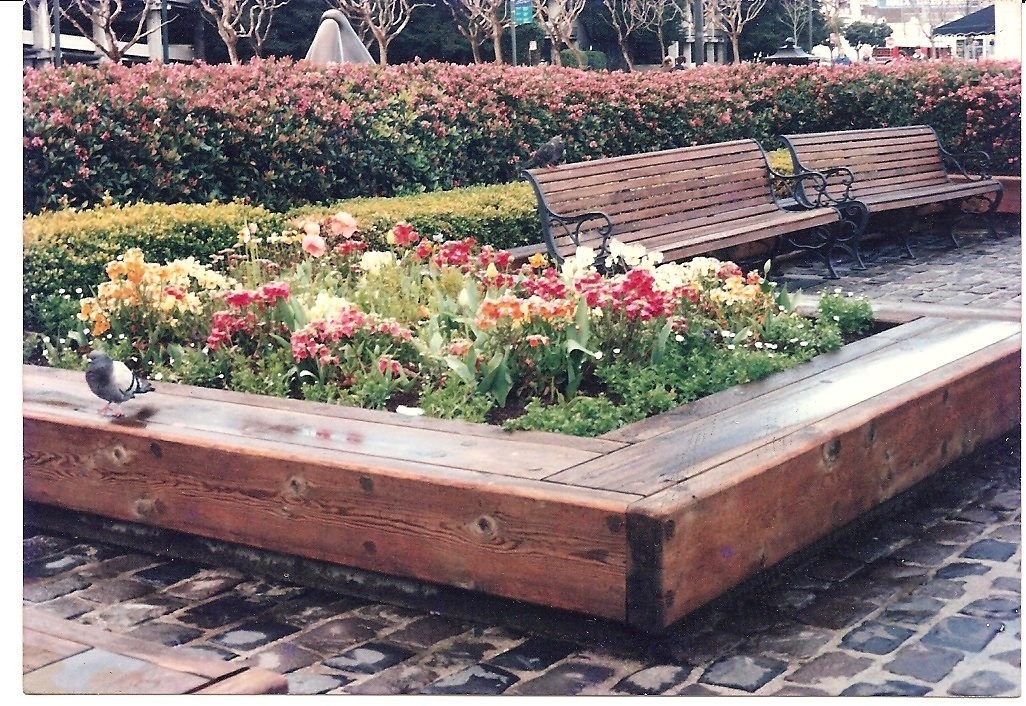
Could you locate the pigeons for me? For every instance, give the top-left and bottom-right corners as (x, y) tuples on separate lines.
(86, 351), (156, 416)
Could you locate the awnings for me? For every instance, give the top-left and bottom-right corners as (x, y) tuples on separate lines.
(932, 5), (995, 39)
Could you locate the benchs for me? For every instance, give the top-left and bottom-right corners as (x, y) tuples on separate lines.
(778, 124), (1005, 271)
(519, 137), (857, 280)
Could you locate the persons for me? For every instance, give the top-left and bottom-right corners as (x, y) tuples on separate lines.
(862, 55), (870, 64)
(909, 45), (928, 61)
(897, 50), (907, 61)
(659, 56), (687, 72)
